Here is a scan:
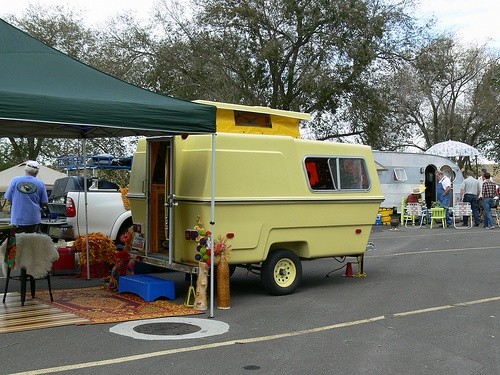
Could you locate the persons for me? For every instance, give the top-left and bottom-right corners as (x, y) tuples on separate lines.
(406, 188), (431, 225)
(3, 161), (48, 234)
(435, 170), (453, 228)
(460, 170), (482, 227)
(478, 168), (492, 197)
(481, 173), (496, 229)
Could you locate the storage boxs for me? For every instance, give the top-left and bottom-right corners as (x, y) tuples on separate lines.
(376, 214), (381, 224)
(54, 248), (75, 271)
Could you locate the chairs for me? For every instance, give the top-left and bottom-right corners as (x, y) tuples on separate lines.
(401, 197), (500, 230)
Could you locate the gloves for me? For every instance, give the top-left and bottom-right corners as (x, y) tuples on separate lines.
(439, 194), (445, 200)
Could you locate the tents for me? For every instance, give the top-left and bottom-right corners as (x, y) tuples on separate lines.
(0, 17), (217, 318)
(0, 160), (69, 192)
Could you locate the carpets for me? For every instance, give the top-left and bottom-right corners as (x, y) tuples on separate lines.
(0, 286), (205, 333)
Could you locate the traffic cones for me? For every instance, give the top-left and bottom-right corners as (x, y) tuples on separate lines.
(184, 285), (196, 308)
(342, 262), (354, 277)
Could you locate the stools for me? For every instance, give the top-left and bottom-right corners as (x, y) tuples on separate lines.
(0, 233), (59, 307)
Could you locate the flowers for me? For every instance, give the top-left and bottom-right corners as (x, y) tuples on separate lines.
(74, 232), (119, 268)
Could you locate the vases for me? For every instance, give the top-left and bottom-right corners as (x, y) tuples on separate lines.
(82, 262), (111, 279)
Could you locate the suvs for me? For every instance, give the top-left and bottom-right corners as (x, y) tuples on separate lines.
(45, 176), (134, 248)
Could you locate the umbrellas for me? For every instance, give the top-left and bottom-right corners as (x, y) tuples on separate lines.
(426, 139), (479, 160)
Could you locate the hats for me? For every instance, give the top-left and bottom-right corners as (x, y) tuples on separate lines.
(411, 188), (423, 195)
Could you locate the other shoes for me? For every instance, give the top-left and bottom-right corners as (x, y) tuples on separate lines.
(475, 223), (479, 226)
(486, 225), (495, 229)
(462, 224), (468, 226)
